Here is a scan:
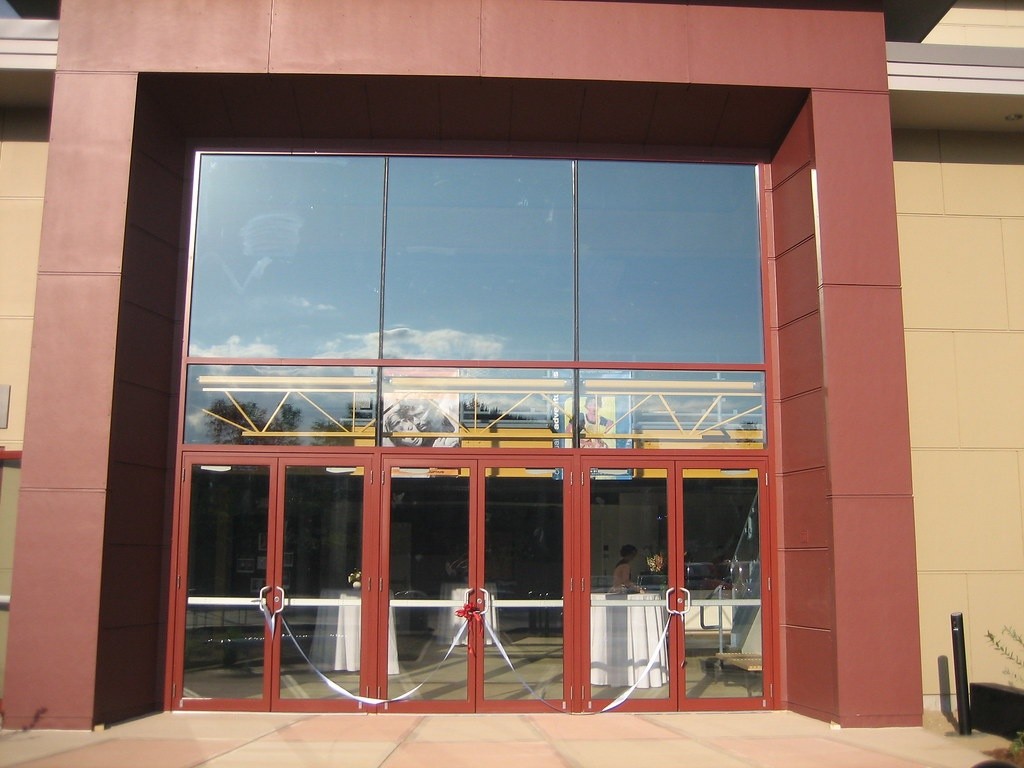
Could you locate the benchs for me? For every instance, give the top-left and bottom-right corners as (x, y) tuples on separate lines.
(715, 653), (762, 697)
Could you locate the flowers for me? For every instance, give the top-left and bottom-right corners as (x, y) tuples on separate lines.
(646, 551), (665, 574)
(344, 570), (364, 591)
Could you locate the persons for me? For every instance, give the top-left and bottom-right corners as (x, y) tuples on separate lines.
(610, 544), (644, 595)
(384, 393), (459, 447)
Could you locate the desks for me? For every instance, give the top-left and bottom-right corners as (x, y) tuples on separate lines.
(305, 588), (400, 675)
(440, 582), (499, 646)
(591, 594), (670, 688)
